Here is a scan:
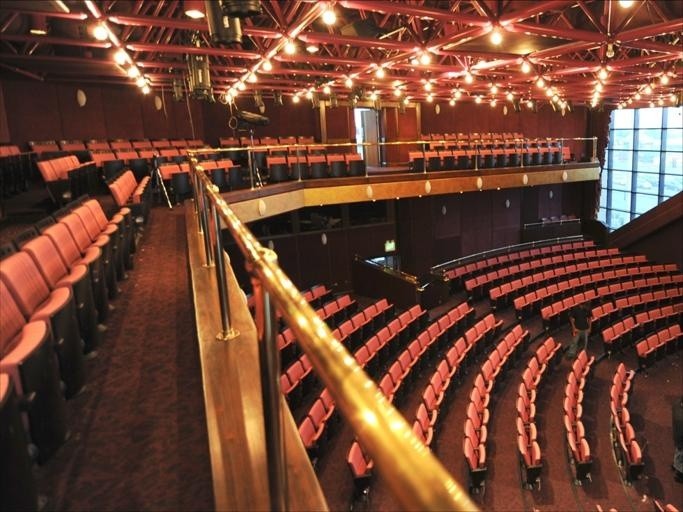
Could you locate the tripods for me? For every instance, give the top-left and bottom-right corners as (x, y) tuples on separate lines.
(143, 154), (173, 211)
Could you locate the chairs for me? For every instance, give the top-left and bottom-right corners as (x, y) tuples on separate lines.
(408, 134), (570, 174)
(2, 145), (153, 512)
(3, 135), (366, 201)
(245, 215), (683, 512)
